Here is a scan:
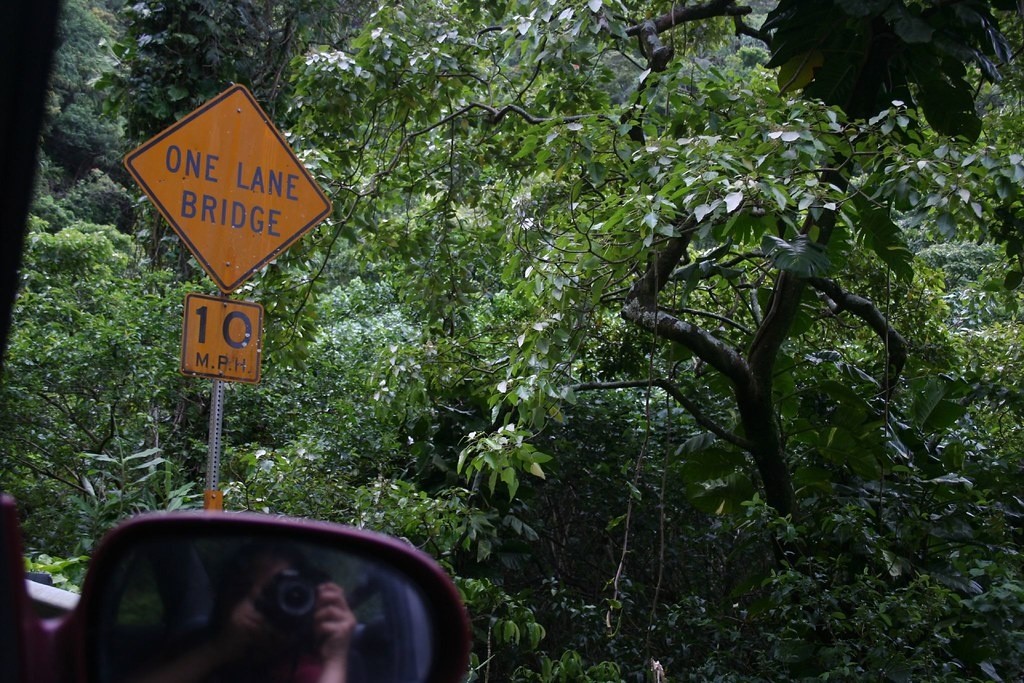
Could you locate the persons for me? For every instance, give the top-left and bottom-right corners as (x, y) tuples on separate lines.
(135, 539), (360, 683)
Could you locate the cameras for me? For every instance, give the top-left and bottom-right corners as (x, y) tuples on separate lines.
(263, 575), (329, 625)
